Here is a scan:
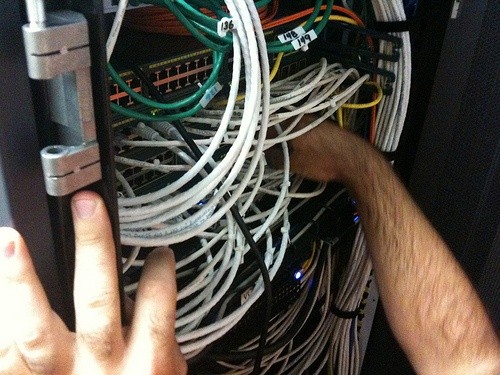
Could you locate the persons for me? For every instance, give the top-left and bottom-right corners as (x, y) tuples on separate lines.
(0, 116), (499, 374)
